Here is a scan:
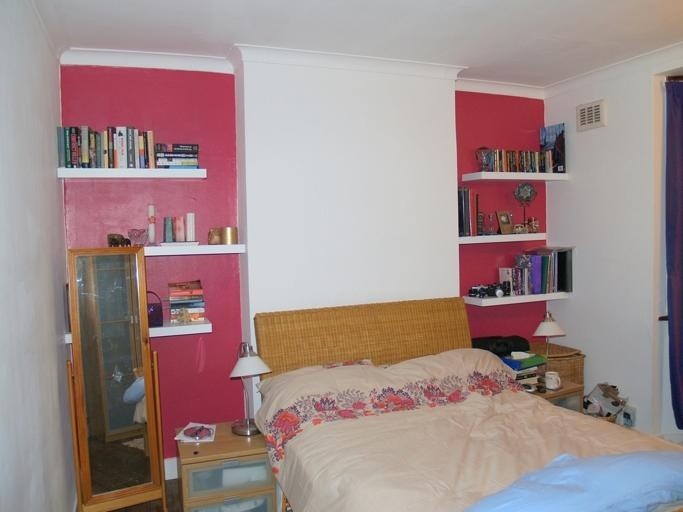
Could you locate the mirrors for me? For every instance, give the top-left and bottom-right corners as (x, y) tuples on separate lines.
(67, 245), (169, 512)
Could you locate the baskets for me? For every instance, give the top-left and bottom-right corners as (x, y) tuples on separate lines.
(530, 343), (585, 385)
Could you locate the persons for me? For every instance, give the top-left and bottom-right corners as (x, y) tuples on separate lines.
(120, 374), (146, 424)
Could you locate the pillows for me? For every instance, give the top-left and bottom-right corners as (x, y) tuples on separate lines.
(256, 358), (379, 400)
(379, 346), (517, 398)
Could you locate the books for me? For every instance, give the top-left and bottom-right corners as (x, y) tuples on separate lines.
(55, 124), (200, 169)
(498, 246), (577, 295)
(167, 278), (205, 325)
(498, 348), (548, 392)
(488, 121), (566, 173)
(457, 184), (479, 237)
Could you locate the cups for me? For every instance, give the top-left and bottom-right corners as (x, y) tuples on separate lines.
(544, 370), (562, 391)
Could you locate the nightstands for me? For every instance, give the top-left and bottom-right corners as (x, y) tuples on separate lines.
(174, 420), (277, 512)
(528, 381), (583, 413)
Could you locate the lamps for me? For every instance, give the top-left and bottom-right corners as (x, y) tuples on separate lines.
(229, 342), (272, 436)
(533, 311), (565, 383)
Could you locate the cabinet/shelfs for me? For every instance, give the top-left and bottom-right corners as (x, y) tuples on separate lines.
(56, 168), (244, 344)
(457, 171), (572, 306)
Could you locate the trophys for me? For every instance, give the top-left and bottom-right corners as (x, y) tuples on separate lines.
(473, 146), (494, 172)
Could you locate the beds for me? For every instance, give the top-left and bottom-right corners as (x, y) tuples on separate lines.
(253, 296), (683, 512)
